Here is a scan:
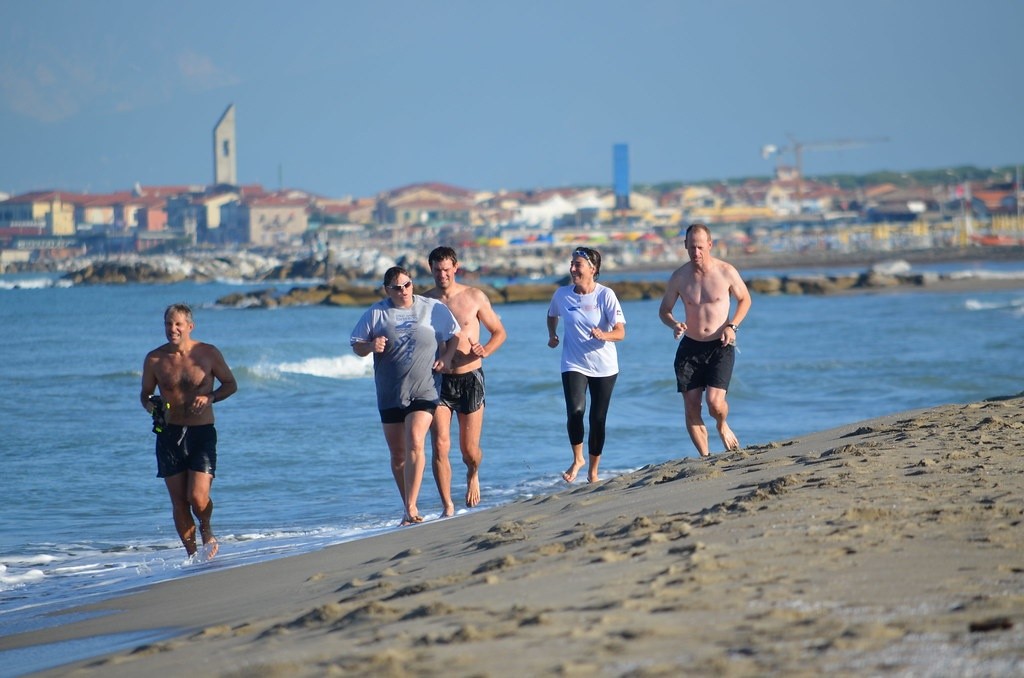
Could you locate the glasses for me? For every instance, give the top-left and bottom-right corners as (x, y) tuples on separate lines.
(572, 251), (593, 267)
(387, 281), (411, 290)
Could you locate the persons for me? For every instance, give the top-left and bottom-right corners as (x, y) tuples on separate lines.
(422, 247), (506, 518)
(547, 246), (626, 484)
(141, 304), (238, 559)
(350, 267), (461, 525)
(658, 225), (752, 457)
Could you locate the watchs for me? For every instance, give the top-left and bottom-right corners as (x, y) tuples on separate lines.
(727, 324), (738, 333)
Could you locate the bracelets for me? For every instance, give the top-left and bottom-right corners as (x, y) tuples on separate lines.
(210, 393), (216, 403)
